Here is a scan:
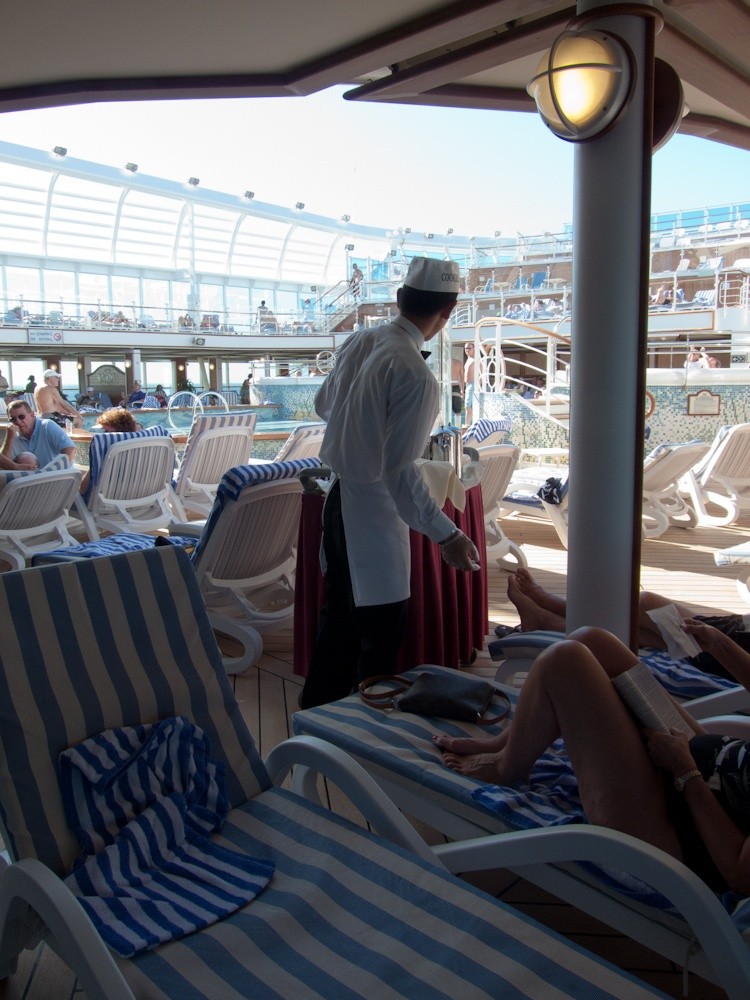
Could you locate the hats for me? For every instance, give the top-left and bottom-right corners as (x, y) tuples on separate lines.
(44, 368), (61, 377)
(405, 256), (461, 292)
(87, 386), (94, 391)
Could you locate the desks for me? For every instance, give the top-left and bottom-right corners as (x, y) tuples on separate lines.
(294, 477), (489, 709)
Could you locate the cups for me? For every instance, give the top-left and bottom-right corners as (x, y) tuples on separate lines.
(65, 418), (72, 433)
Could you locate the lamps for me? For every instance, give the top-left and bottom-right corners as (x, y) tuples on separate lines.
(310, 286), (316, 291)
(245, 191), (254, 198)
(343, 214), (350, 221)
(126, 163), (138, 171)
(391, 250), (396, 255)
(448, 229), (453, 233)
(527, 29), (637, 142)
(296, 203), (304, 208)
(189, 177), (199, 185)
(406, 227), (411, 232)
(345, 244), (354, 250)
(427, 233), (434, 238)
(494, 230), (500, 236)
(55, 147), (67, 154)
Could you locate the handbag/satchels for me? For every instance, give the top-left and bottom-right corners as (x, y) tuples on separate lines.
(358, 672), (513, 724)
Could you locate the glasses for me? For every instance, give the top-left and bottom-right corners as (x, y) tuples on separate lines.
(9, 412), (28, 423)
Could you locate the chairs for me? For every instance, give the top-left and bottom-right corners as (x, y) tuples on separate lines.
(0, 257), (750, 1000)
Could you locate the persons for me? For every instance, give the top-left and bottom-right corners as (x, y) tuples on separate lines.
(256, 301), (278, 332)
(0, 369), (9, 398)
(463, 342), (477, 425)
(348, 264), (365, 305)
(300, 257), (480, 709)
(682, 348), (715, 369)
(125, 382), (146, 408)
(239, 374), (252, 405)
(77, 387), (102, 413)
(78, 408), (143, 504)
(0, 400), (77, 471)
(505, 377), (545, 398)
(504, 299), (562, 320)
(26, 376), (39, 394)
(154, 385), (171, 407)
(649, 284), (685, 305)
(435, 566), (750, 912)
(34, 369), (84, 431)
